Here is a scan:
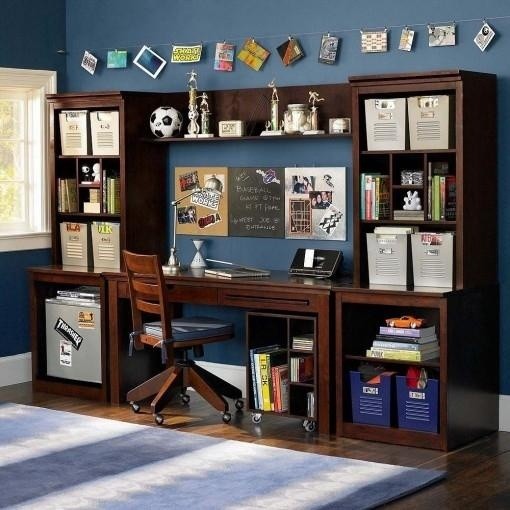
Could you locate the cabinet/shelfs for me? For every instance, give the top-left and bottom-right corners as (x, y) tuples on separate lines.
(333, 287), (498, 451)
(26, 90), (161, 264)
(27, 266), (110, 404)
(244, 311), (320, 433)
(348, 69), (500, 293)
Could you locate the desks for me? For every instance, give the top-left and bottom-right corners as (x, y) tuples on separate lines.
(102, 267), (350, 439)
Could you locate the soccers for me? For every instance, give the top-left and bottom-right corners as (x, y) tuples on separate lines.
(150, 106), (183, 138)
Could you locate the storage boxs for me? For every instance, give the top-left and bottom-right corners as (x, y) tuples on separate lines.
(398, 374), (440, 433)
(351, 372), (396, 427)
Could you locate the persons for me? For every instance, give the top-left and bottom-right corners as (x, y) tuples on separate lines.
(311, 191), (332, 209)
(303, 177), (313, 194)
(293, 177), (305, 194)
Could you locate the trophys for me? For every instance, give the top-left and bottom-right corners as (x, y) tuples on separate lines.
(303, 90), (325, 135)
(184, 69), (214, 139)
(260, 77), (285, 136)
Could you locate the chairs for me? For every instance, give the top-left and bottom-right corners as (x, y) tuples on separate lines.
(119, 249), (243, 424)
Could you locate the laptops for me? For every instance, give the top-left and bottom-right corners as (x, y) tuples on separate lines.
(205, 258), (271, 277)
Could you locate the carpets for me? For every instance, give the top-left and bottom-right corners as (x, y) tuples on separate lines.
(0, 400), (448, 510)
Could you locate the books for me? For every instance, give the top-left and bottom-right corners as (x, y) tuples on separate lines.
(430, 175), (457, 221)
(57, 178), (77, 212)
(103, 169), (121, 215)
(57, 286), (100, 303)
(250, 335), (315, 418)
(366, 326), (439, 362)
(360, 172), (389, 220)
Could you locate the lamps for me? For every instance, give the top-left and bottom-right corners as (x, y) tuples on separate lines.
(157, 178), (223, 271)
